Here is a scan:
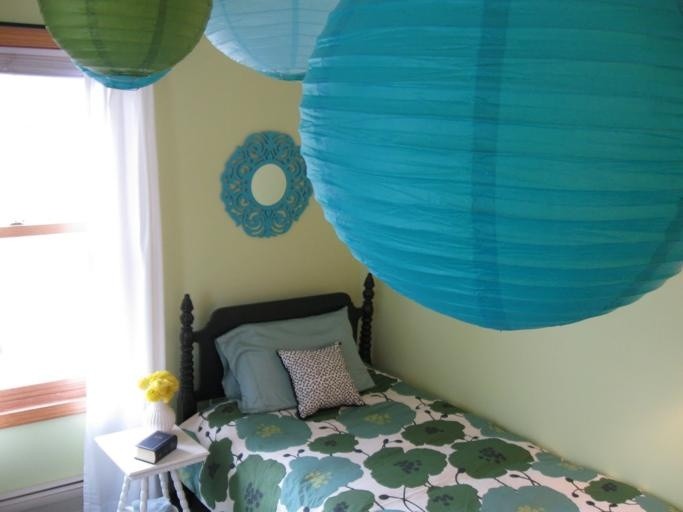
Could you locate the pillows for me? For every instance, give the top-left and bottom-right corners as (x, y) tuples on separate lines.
(277, 343), (365, 419)
(215, 306), (376, 414)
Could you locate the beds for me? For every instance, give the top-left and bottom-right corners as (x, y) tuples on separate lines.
(179, 273), (683, 512)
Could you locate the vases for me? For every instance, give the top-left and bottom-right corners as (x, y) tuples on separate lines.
(146, 400), (175, 433)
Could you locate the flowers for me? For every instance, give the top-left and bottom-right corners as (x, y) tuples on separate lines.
(139, 370), (181, 404)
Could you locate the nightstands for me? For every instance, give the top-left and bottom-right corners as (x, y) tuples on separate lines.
(94, 425), (210, 512)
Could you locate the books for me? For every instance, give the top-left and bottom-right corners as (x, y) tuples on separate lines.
(134, 430), (178, 464)
